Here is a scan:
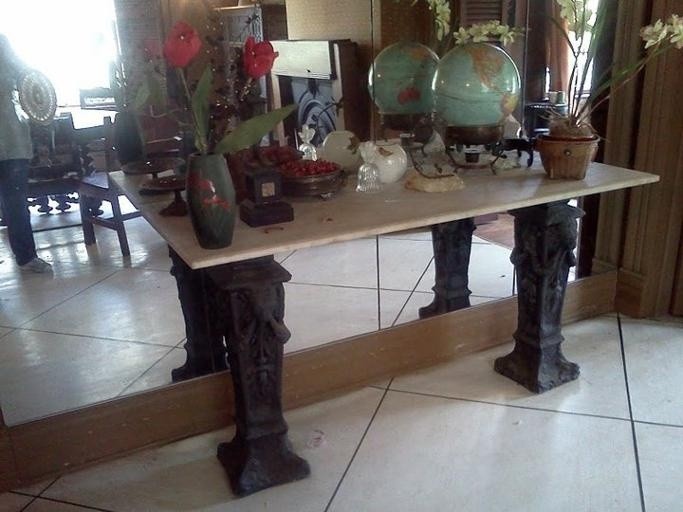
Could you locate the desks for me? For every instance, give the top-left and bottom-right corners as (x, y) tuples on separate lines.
(137, 139), (661, 500)
(104, 134), (484, 386)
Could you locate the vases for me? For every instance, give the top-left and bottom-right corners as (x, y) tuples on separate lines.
(537, 122), (597, 180)
(188, 149), (240, 253)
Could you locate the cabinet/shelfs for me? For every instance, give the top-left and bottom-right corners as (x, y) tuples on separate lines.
(211, 1), (285, 149)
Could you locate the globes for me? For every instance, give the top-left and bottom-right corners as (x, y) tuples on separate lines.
(432, 42), (521, 175)
(367, 41), (440, 158)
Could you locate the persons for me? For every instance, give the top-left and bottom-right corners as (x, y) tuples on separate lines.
(1, 35), (55, 278)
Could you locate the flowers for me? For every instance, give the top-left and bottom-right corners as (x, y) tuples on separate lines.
(535, 0), (682, 131)
(156, 18), (305, 158)
(425, 0), (529, 58)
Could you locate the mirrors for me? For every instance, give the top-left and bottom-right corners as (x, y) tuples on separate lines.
(0, 0), (621, 436)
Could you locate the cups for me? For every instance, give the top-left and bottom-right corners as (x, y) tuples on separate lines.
(465, 150), (480, 163)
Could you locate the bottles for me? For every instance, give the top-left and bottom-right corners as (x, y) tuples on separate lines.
(557, 91), (566, 104)
(548, 92), (556, 103)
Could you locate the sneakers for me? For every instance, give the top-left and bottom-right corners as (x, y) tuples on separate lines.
(16, 256), (55, 273)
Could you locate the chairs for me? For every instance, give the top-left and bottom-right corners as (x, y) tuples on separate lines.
(77, 110), (149, 263)
(15, 106), (97, 250)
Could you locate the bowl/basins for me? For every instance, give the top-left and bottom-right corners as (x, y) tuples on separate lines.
(278, 160), (342, 198)
